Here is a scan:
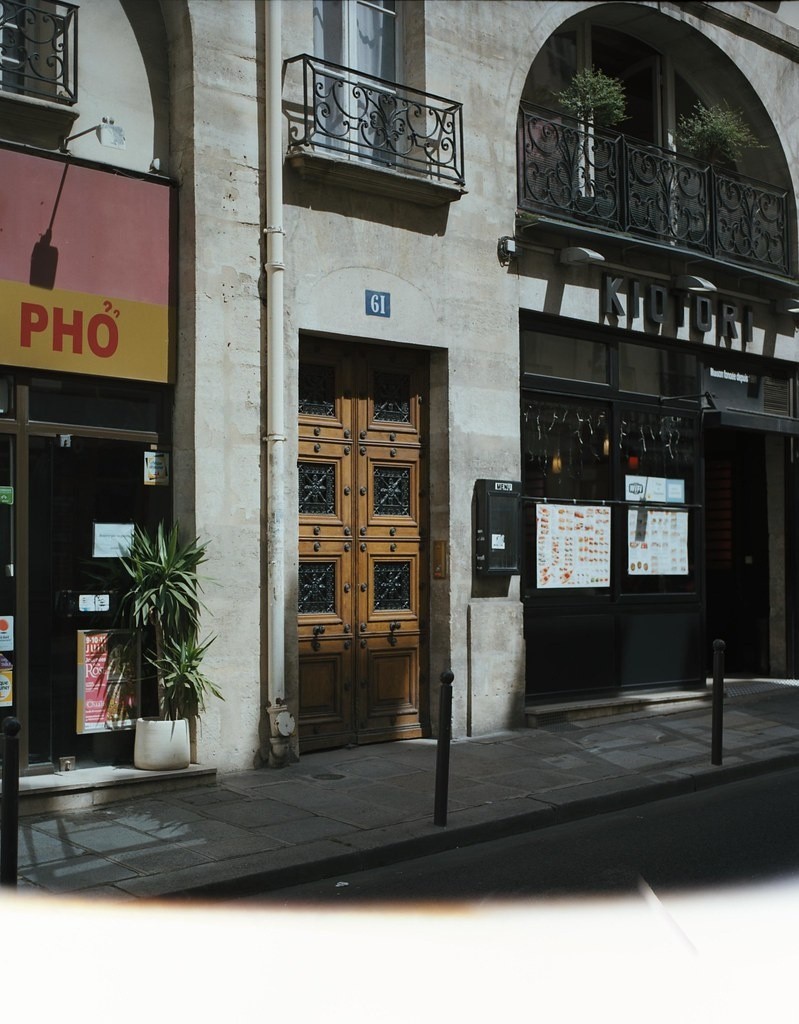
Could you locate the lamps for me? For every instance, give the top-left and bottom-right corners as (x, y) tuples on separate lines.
(671, 273), (717, 292)
(560, 247), (605, 266)
(57, 118), (126, 154)
(552, 437), (561, 474)
(629, 444), (639, 470)
(658, 390), (722, 428)
(777, 297), (799, 317)
(604, 431), (610, 456)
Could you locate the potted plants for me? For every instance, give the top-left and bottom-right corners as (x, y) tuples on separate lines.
(115, 518), (225, 771)
(547, 64), (771, 254)
